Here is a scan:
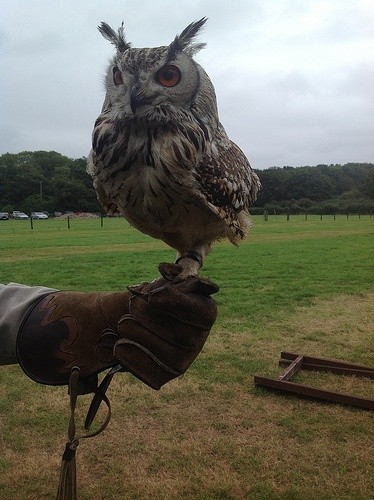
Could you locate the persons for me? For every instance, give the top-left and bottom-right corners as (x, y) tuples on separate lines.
(0, 261), (221, 389)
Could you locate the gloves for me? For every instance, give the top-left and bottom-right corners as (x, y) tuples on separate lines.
(18, 272), (220, 499)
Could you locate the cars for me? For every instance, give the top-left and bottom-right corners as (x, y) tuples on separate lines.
(15, 213), (28, 220)
(10, 211), (20, 218)
(32, 212), (48, 219)
(0, 213), (9, 220)
